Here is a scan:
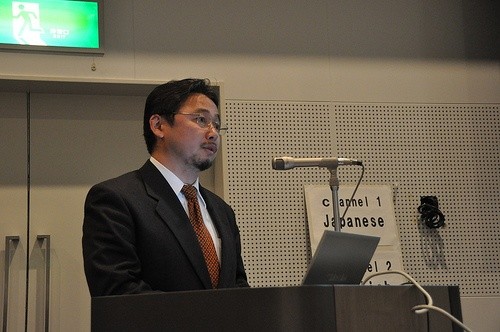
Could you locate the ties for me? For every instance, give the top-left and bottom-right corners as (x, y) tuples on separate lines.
(181, 185), (220, 289)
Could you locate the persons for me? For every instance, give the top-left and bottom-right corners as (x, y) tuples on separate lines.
(81, 78), (251, 297)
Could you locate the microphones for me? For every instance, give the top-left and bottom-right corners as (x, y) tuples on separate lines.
(272, 157), (363, 170)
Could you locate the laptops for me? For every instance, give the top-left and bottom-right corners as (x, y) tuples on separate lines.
(300, 231), (380, 286)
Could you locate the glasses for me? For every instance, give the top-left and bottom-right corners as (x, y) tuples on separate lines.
(173, 112), (228, 137)
